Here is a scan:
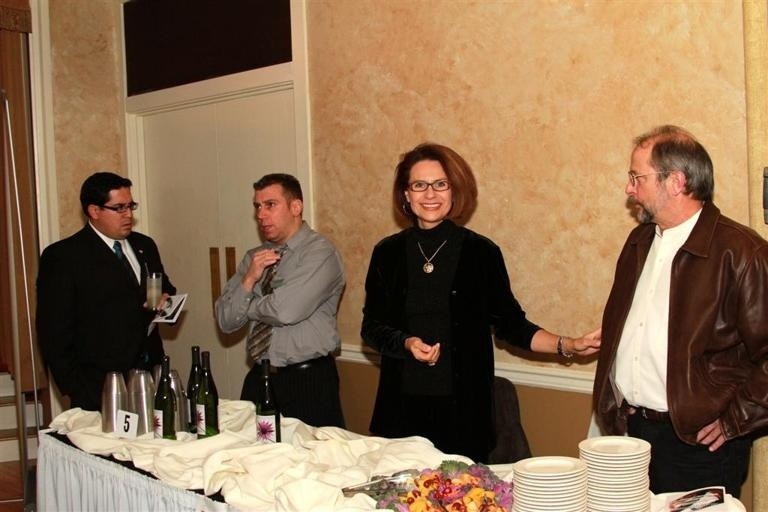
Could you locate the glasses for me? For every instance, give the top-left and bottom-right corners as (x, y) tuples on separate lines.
(628, 171), (663, 185)
(104, 202), (138, 212)
(409, 180), (451, 191)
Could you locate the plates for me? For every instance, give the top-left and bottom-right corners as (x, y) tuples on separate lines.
(577, 435), (652, 512)
(510, 455), (587, 512)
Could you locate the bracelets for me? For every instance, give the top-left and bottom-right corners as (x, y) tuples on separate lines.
(557, 337), (574, 360)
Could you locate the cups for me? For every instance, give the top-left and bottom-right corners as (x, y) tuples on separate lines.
(145, 272), (163, 309)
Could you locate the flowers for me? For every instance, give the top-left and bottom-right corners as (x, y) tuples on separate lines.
(341, 460), (513, 511)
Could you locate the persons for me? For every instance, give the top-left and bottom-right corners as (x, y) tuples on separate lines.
(37, 171), (177, 409)
(364, 143), (602, 465)
(589, 124), (768, 497)
(213, 172), (346, 430)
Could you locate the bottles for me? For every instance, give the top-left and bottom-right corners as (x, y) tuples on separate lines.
(187, 345), (219, 440)
(154, 355), (177, 441)
(256, 358), (281, 444)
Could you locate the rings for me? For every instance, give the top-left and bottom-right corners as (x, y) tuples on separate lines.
(427, 361), (435, 368)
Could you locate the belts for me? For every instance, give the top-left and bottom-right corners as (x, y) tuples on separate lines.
(634, 406), (668, 419)
(280, 358), (320, 370)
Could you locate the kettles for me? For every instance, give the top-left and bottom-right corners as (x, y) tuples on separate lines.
(101, 372), (128, 433)
(167, 370), (188, 430)
(127, 369), (155, 436)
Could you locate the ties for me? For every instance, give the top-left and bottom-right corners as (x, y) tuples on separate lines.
(247, 252), (283, 363)
(114, 241), (139, 288)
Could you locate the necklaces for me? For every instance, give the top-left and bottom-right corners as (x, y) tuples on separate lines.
(416, 239), (449, 274)
